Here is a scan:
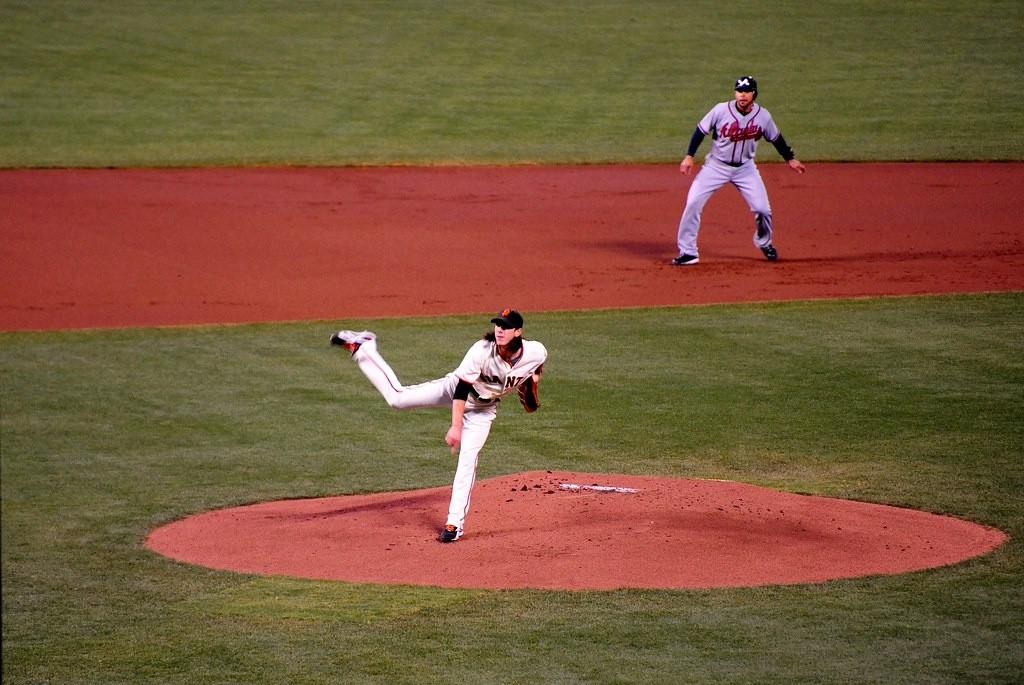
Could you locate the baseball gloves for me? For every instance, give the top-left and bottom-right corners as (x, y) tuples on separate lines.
(516, 380), (542, 414)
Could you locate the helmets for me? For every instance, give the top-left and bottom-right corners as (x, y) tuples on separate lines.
(735, 76), (757, 90)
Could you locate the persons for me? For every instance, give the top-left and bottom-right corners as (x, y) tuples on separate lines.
(672, 76), (806, 266)
(330, 308), (548, 543)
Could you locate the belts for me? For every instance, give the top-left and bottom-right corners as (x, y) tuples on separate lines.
(723, 161), (744, 167)
(470, 386), (494, 403)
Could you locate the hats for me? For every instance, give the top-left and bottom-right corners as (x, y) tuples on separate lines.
(491, 309), (523, 328)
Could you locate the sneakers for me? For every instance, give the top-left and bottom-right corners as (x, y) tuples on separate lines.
(672, 254), (699, 265)
(330, 330), (377, 356)
(440, 525), (464, 541)
(760, 244), (777, 262)
